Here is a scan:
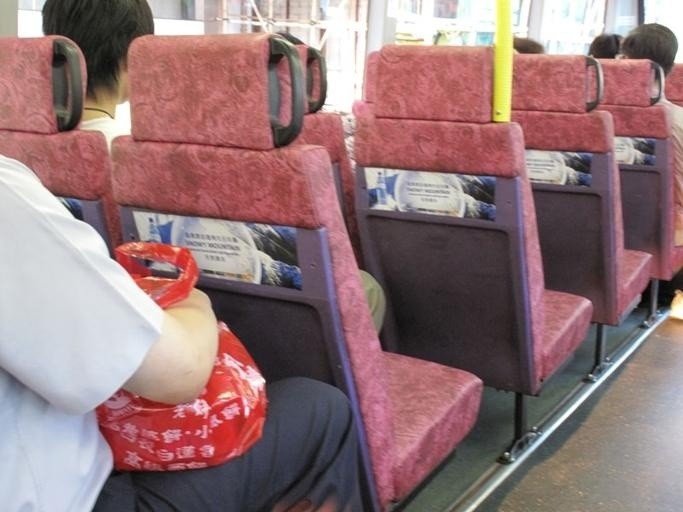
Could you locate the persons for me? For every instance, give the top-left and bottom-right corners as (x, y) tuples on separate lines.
(41, 0), (155, 155)
(513, 37), (544, 54)
(588, 34), (624, 58)
(0, 154), (364, 512)
(623, 23), (683, 320)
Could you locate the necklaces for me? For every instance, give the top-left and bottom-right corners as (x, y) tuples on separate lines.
(83, 107), (115, 120)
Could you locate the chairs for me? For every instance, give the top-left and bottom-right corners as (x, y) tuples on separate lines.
(287, 43), (597, 464)
(0, 31), (484, 510)
(510, 52), (682, 382)
